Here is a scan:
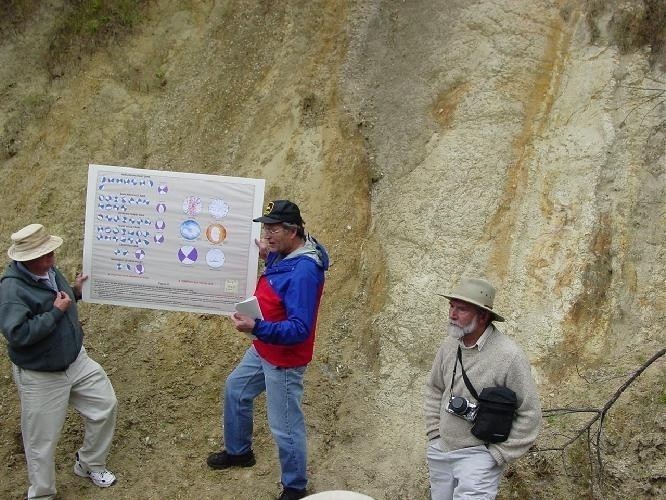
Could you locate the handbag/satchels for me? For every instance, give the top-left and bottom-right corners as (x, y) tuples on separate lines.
(470, 387), (516, 443)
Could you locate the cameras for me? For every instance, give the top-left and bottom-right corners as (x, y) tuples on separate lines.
(444, 395), (480, 422)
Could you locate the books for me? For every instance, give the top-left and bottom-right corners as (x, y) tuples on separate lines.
(234, 295), (266, 342)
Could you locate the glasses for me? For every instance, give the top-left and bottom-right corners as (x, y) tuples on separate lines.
(262, 226), (285, 236)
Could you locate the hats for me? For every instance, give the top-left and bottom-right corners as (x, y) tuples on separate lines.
(437, 277), (506, 322)
(7, 224), (63, 262)
(253, 200), (301, 224)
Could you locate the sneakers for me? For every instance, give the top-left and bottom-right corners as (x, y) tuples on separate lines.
(207, 449), (255, 469)
(73, 452), (116, 487)
(278, 488), (306, 500)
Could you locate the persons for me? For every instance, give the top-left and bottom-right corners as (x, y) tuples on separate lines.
(205, 199), (330, 499)
(1, 222), (119, 500)
(421, 277), (542, 500)
